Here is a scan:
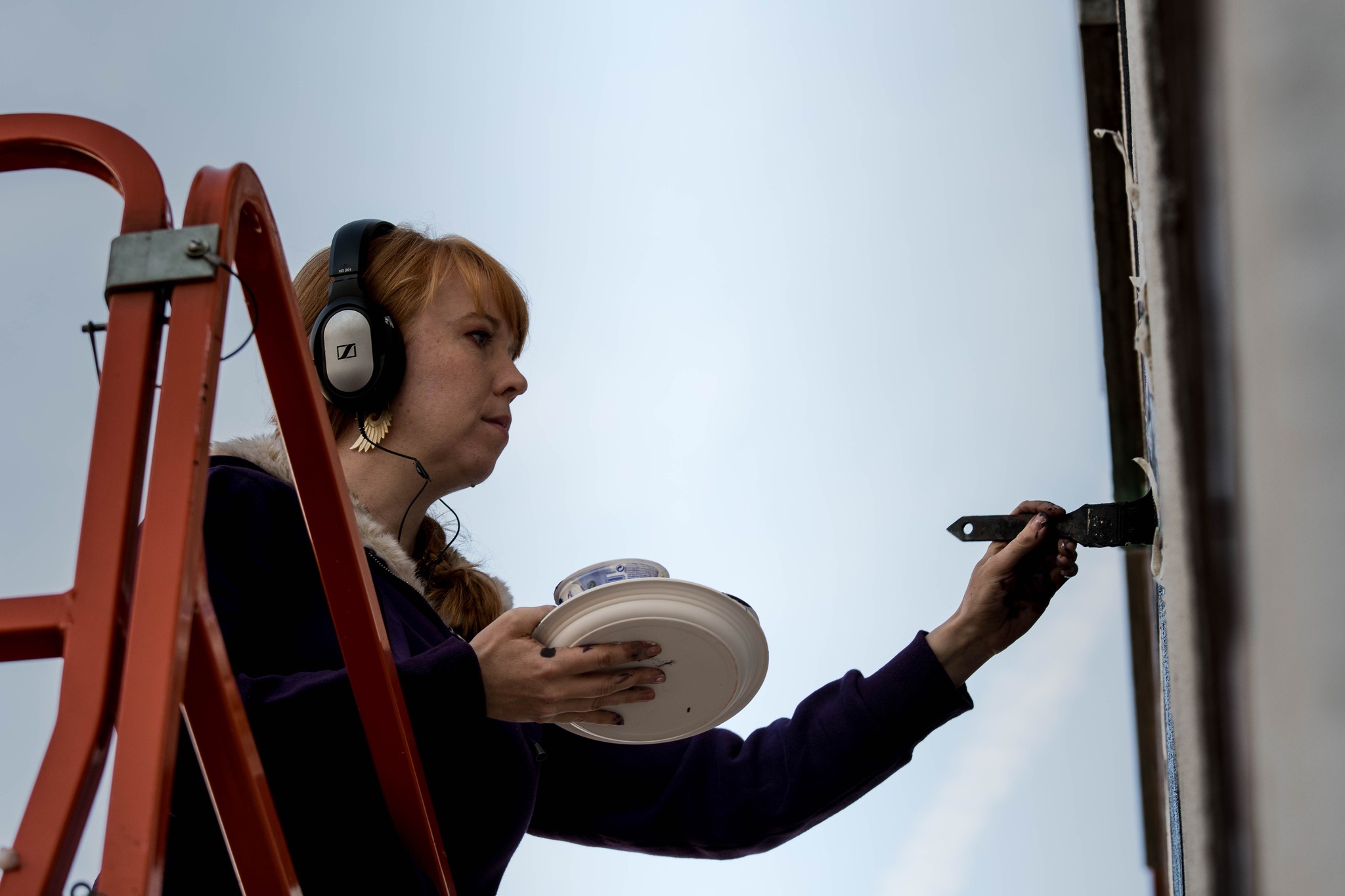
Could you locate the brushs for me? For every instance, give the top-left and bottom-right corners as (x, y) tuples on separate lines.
(946, 487), (1159, 547)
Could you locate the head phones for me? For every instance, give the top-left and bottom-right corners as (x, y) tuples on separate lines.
(305, 220), (411, 411)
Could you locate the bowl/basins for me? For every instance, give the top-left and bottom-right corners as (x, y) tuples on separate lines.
(553, 558), (670, 605)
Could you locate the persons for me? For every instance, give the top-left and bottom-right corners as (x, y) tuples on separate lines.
(162, 220), (1081, 896)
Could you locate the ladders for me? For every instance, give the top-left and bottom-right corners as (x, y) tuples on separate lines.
(0, 107), (455, 896)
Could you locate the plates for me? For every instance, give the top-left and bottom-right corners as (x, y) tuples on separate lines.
(530, 577), (769, 745)
(722, 592), (760, 623)
